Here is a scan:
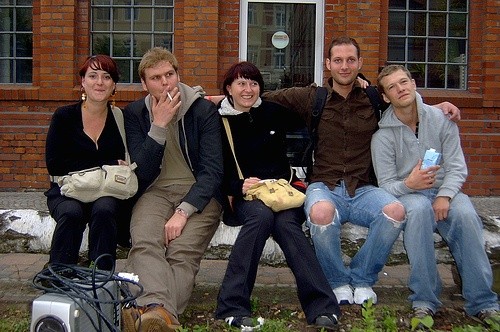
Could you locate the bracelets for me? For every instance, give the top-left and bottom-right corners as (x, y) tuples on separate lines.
(175, 208), (189, 219)
(208, 96), (211, 101)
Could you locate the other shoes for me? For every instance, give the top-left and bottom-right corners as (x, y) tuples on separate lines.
(134, 303), (180, 332)
(412, 306), (434, 330)
(476, 310), (500, 330)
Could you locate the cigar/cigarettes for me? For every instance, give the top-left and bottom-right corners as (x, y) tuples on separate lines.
(167, 91), (173, 101)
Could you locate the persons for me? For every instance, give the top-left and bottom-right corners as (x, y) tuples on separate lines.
(123, 46), (224, 332)
(214, 61), (341, 332)
(371, 64), (500, 329)
(43, 54), (126, 271)
(204, 36), (461, 306)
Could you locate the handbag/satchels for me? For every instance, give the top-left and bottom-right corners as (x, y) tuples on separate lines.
(59, 161), (139, 204)
(245, 178), (306, 213)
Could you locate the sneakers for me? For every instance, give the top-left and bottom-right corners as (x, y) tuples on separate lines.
(353, 286), (378, 306)
(332, 283), (354, 306)
(223, 315), (255, 330)
(312, 313), (341, 331)
